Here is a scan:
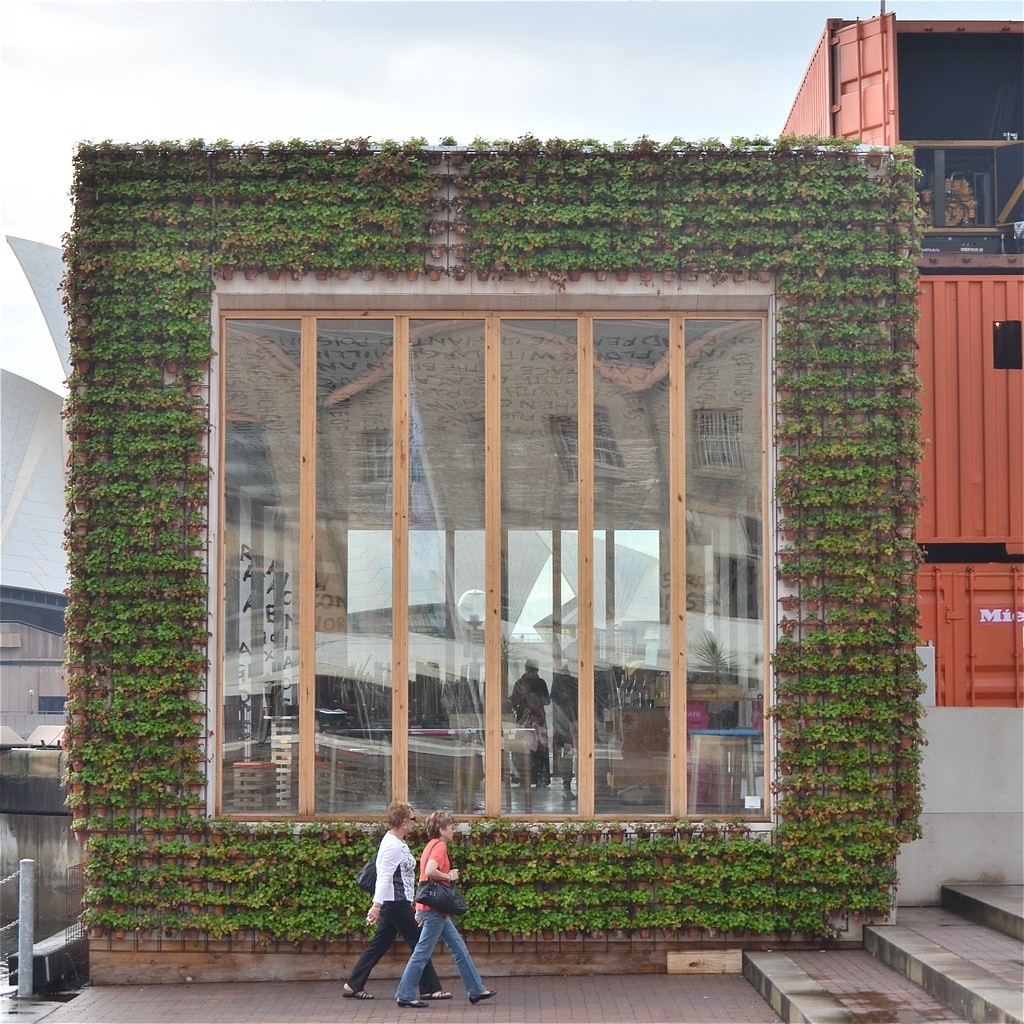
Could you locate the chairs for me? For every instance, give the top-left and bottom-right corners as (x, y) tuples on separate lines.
(460, 680), (762, 817)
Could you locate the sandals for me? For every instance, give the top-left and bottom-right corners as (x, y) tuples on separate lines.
(420, 991), (452, 999)
(342, 983), (374, 999)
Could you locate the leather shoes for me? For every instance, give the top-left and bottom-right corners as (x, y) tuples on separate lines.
(397, 997), (429, 1007)
(469, 990), (498, 1005)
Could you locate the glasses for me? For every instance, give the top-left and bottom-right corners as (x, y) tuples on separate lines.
(409, 816), (416, 821)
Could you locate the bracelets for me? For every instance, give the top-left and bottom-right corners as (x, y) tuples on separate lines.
(369, 906), (381, 914)
(448, 873), (452, 881)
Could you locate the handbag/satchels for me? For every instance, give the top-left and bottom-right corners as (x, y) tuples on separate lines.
(356, 855), (376, 895)
(415, 841), (467, 915)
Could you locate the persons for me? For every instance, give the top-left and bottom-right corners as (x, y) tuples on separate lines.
(551, 661), (608, 802)
(394, 809), (498, 1009)
(510, 657), (551, 787)
(342, 800), (453, 999)
